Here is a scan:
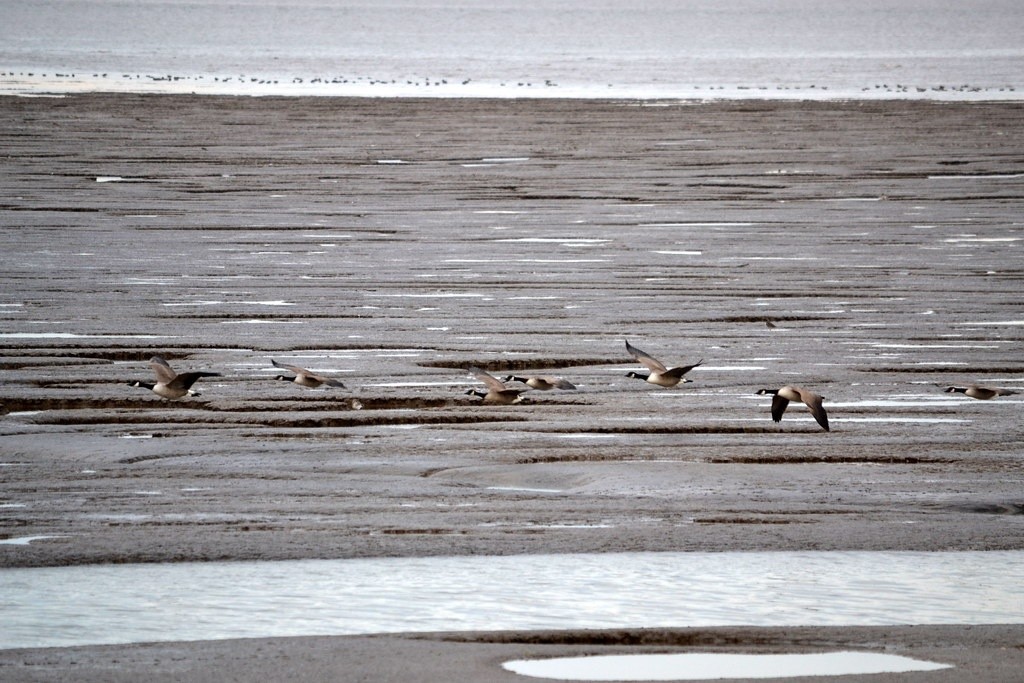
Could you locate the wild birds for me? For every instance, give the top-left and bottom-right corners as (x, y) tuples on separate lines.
(754, 386), (829, 432)
(464, 364), (530, 404)
(623, 338), (706, 387)
(504, 375), (577, 391)
(126, 356), (225, 401)
(944, 386), (1021, 399)
(271, 359), (347, 390)
